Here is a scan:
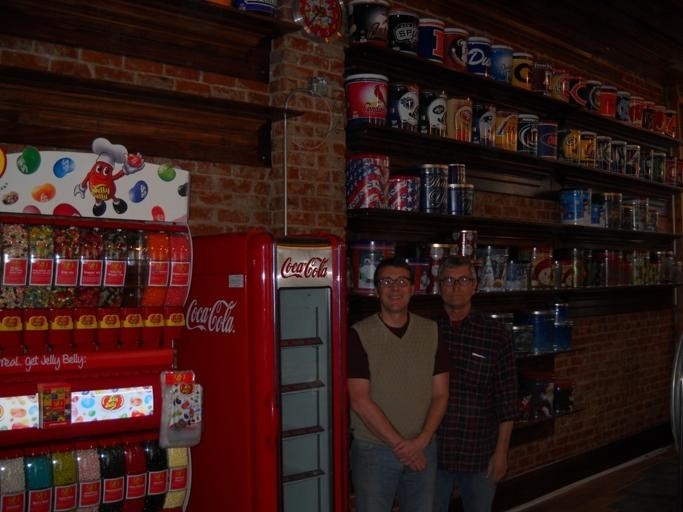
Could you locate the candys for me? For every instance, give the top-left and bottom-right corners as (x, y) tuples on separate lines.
(0, 440), (188, 512)
(0, 224), (190, 308)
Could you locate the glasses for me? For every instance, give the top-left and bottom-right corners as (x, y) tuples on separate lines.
(376, 276), (413, 287)
(435, 276), (479, 287)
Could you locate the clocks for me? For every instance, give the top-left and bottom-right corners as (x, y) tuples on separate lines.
(289, 1), (350, 46)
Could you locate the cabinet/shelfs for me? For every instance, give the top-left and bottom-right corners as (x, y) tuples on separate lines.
(341, 39), (683, 322)
(505, 347), (590, 452)
(279, 338), (327, 484)
(0, 1), (307, 182)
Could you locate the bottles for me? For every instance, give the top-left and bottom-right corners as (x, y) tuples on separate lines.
(0, 214), (191, 312)
(0, 428), (194, 511)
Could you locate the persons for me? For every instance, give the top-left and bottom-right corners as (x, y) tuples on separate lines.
(347, 256), (450, 512)
(432, 256), (519, 512)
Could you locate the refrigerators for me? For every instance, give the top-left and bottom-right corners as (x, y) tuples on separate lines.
(165, 230), (348, 510)
